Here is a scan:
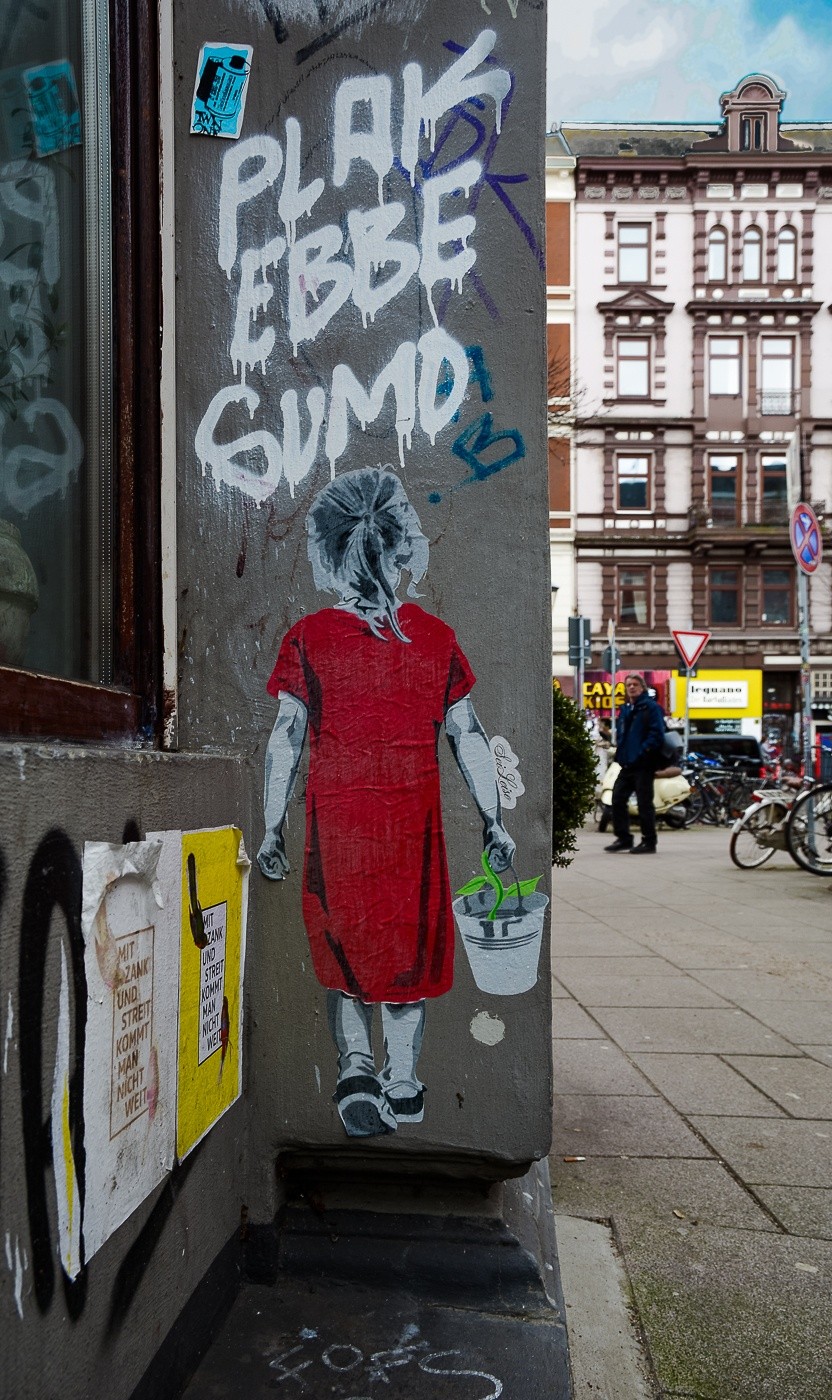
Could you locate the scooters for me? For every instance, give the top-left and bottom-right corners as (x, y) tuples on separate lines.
(596, 743), (691, 831)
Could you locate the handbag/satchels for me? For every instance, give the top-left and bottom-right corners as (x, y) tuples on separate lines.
(655, 731), (683, 771)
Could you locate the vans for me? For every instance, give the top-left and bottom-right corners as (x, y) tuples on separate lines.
(682, 732), (765, 778)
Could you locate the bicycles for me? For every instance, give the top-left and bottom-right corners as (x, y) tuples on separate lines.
(592, 741), (832, 878)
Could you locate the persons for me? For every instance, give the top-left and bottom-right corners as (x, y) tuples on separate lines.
(599, 718), (612, 741)
(759, 737), (776, 760)
(603, 672), (665, 854)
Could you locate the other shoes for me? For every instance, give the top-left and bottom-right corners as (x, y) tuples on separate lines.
(603, 837), (633, 851)
(629, 843), (656, 854)
